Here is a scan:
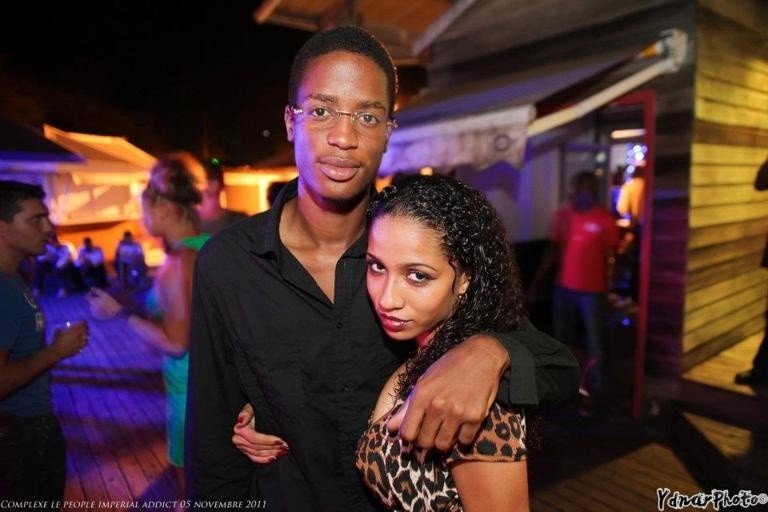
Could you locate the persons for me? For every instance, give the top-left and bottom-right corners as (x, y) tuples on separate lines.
(526, 172), (621, 417)
(232, 174), (541, 512)
(183, 23), (581, 512)
(0, 151), (248, 512)
(735, 160), (768, 386)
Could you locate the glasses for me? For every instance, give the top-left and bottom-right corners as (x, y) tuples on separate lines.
(289, 105), (389, 138)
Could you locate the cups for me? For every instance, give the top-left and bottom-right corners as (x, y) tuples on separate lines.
(57, 319), (80, 332)
